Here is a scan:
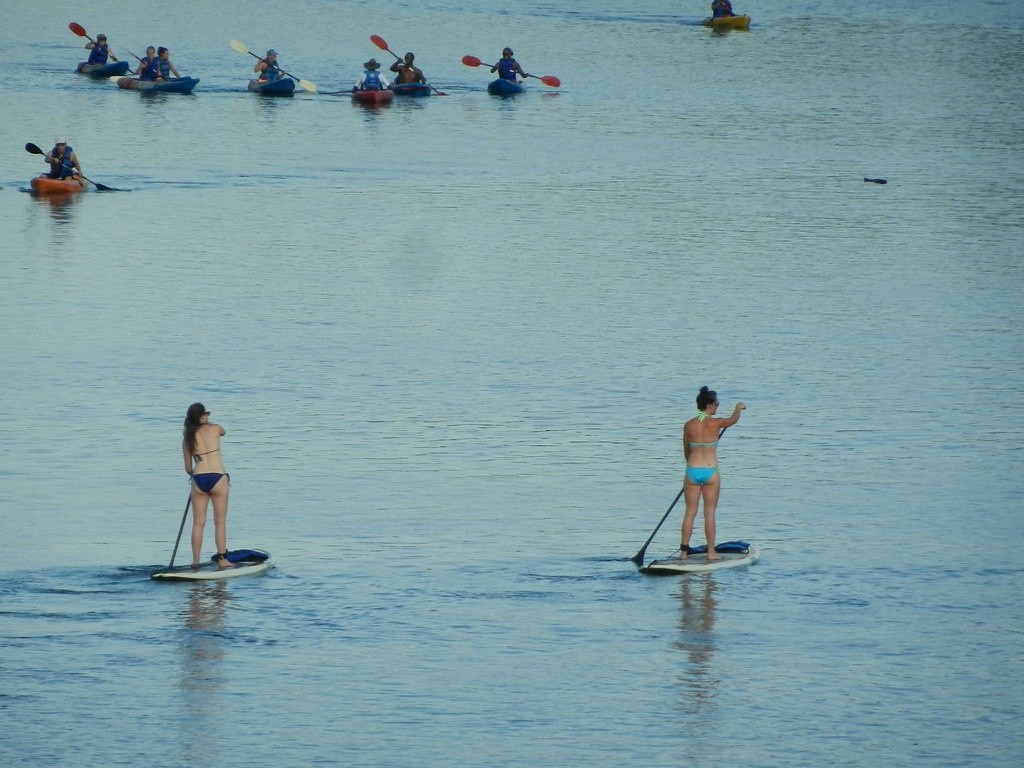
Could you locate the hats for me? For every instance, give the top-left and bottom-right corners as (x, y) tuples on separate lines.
(364, 59), (381, 70)
(55, 136), (66, 146)
(267, 49), (279, 56)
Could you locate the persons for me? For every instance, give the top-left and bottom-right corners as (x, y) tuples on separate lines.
(254, 49), (287, 84)
(390, 52), (427, 85)
(39, 135), (83, 180)
(680, 385), (746, 559)
(182, 403), (235, 568)
(712, 0), (737, 17)
(490, 47), (529, 84)
(135, 46), (180, 81)
(85, 34), (118, 65)
(351, 59), (396, 94)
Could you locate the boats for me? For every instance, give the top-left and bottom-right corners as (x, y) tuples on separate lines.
(31, 172), (86, 195)
(247, 77), (296, 95)
(351, 88), (395, 105)
(702, 14), (753, 30)
(488, 79), (526, 97)
(116, 77), (202, 94)
(389, 83), (432, 98)
(76, 60), (130, 78)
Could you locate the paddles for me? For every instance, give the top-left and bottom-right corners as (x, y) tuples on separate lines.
(25, 142), (113, 191)
(370, 34), (448, 96)
(324, 85), (424, 95)
(68, 21), (133, 73)
(632, 426), (728, 564)
(166, 493), (192, 568)
(230, 39), (317, 92)
(123, 46), (170, 81)
(462, 55), (561, 88)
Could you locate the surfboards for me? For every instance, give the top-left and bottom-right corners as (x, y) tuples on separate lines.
(639, 541), (761, 574)
(151, 546), (279, 583)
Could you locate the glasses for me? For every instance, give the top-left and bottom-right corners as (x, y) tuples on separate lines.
(56, 143), (64, 147)
(710, 402), (719, 407)
(203, 411), (210, 416)
